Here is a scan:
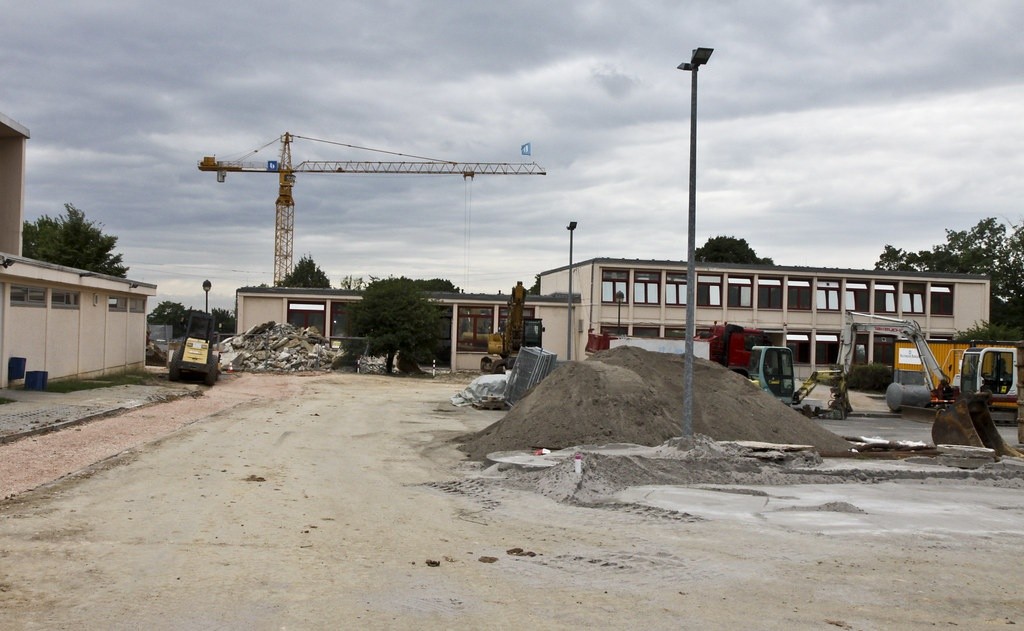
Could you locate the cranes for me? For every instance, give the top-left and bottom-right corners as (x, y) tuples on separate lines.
(196, 131), (546, 289)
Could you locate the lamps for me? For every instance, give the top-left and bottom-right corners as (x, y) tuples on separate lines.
(129, 281), (139, 289)
(2, 258), (16, 269)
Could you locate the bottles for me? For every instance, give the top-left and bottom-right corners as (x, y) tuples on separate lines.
(575, 453), (581, 473)
(227, 361), (234, 373)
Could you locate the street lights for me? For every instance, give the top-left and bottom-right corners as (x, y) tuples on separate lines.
(565, 220), (578, 360)
(677, 46), (715, 452)
(201, 279), (212, 315)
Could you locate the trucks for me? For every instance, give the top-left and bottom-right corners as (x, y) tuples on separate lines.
(584, 321), (775, 381)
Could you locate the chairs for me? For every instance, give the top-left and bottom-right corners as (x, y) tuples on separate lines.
(995, 358), (1009, 379)
(772, 367), (781, 374)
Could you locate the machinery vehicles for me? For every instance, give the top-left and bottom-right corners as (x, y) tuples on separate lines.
(168, 311), (221, 387)
(899, 346), (1017, 424)
(750, 345), (853, 420)
(479, 280), (546, 375)
